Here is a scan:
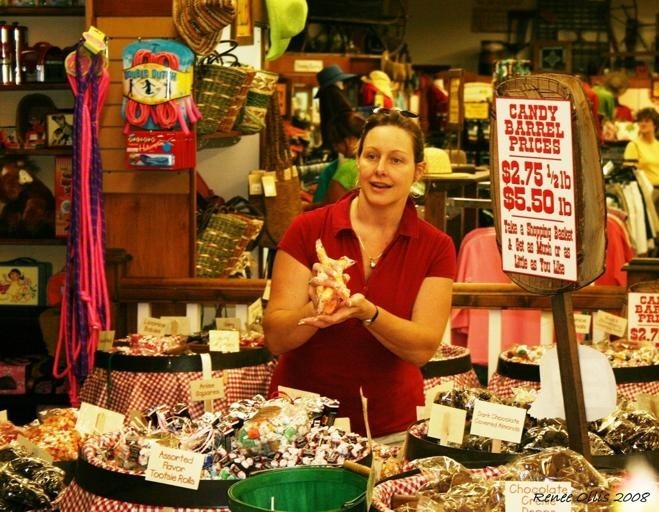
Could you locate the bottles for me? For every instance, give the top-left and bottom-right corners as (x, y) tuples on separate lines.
(0, 20), (28, 86)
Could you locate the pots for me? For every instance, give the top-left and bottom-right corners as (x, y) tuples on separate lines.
(20, 42), (60, 84)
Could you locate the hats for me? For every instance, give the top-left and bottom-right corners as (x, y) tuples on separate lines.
(369, 71), (392, 100)
(314, 65), (356, 98)
(173, 0), (236, 57)
(423, 148), (467, 179)
(267, 0), (309, 62)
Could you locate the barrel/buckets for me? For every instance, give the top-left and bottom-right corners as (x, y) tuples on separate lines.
(226, 467), (369, 512)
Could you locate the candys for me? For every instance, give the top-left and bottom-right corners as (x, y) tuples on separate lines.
(316, 238), (357, 315)
(507, 342), (659, 368)
(0, 394), (428, 480)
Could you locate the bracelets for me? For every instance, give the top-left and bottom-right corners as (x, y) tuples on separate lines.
(363, 306), (379, 327)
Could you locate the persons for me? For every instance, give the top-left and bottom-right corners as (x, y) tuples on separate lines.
(623, 107), (659, 188)
(427, 77), (450, 145)
(300, 110), (368, 211)
(262, 109), (458, 455)
(51, 115), (73, 145)
(591, 81), (614, 119)
(604, 73), (634, 122)
(577, 74), (603, 146)
(313, 65), (359, 143)
(2, 268), (25, 302)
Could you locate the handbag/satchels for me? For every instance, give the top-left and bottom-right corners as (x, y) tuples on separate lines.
(249, 167), (301, 249)
(21, 46), (66, 87)
(197, 41), (279, 136)
(195, 195), (267, 277)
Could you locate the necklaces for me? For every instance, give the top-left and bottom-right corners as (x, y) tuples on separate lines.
(357, 232), (384, 268)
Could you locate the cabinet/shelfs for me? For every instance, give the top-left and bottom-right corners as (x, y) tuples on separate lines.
(1, 0), (96, 408)
